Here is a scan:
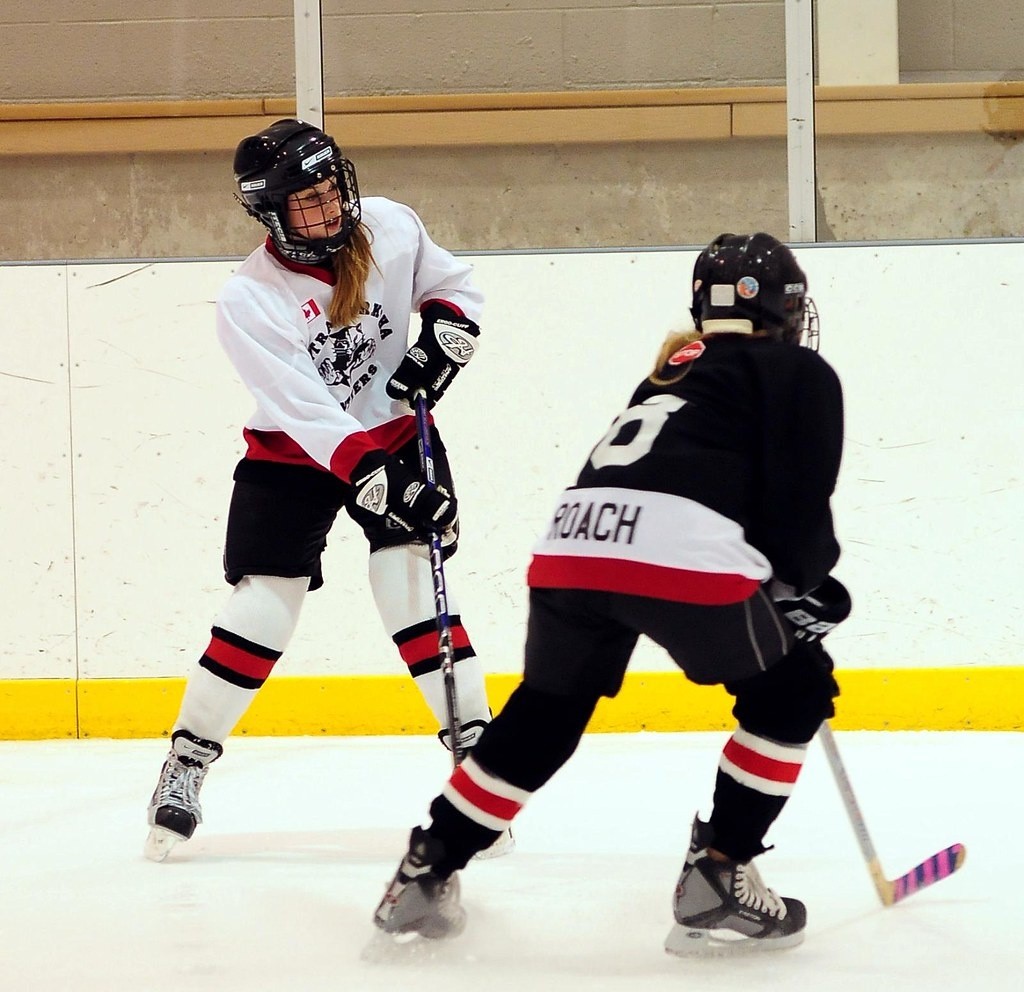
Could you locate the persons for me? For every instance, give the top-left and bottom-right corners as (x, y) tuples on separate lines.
(960, 68), (1024, 236)
(143, 119), (513, 862)
(366, 234), (852, 958)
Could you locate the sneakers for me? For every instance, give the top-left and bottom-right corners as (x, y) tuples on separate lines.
(144, 729), (223, 863)
(664, 812), (807, 956)
(361, 824), (466, 959)
(438, 706), (519, 859)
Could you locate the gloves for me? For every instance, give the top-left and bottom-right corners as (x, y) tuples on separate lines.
(349, 448), (457, 538)
(386, 298), (481, 411)
(775, 576), (850, 643)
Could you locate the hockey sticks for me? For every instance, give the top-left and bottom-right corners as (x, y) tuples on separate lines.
(412, 385), (465, 768)
(819, 720), (967, 907)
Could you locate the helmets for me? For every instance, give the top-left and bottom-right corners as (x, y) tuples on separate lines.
(688, 232), (821, 351)
(231, 118), (362, 265)
